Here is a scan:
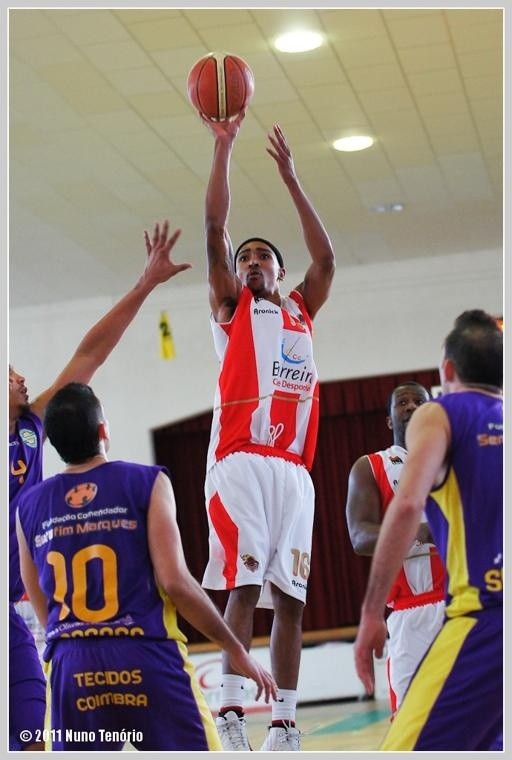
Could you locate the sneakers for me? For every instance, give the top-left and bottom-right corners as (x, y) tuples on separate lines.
(215, 711), (252, 749)
(261, 726), (299, 751)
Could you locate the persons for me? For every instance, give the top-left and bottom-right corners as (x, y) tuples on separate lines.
(353, 309), (503, 751)
(15, 382), (279, 751)
(9, 218), (195, 751)
(345, 381), (450, 724)
(198, 105), (337, 751)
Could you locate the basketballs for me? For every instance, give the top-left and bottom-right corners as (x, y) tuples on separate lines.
(188, 52), (253, 118)
